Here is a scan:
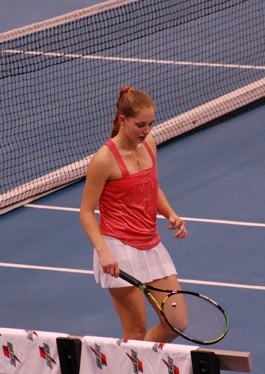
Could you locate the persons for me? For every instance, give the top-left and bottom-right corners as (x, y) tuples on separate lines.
(80, 86), (189, 344)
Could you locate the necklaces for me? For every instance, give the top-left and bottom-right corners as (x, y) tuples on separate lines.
(116, 136), (141, 167)
